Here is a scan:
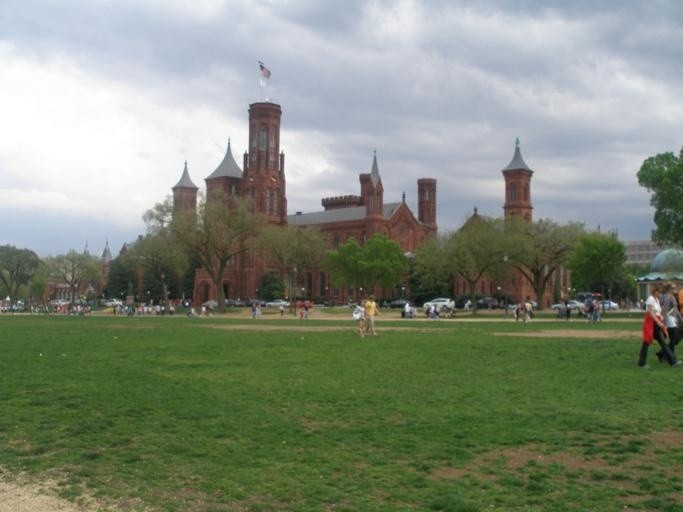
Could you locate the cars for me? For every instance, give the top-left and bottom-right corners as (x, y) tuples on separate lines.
(202, 299), (313, 309)
(383, 298), (412, 307)
(550, 300), (584, 311)
(598, 300), (619, 310)
(507, 301), (536, 309)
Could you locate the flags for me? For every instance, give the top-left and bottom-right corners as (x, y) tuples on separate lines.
(259, 61), (271, 79)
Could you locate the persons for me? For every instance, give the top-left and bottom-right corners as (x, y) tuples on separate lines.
(654, 278), (683, 363)
(255, 303), (262, 318)
(1, 296), (211, 320)
(512, 295), (604, 326)
(279, 304), (286, 319)
(402, 302), (456, 320)
(303, 307), (309, 319)
(363, 294), (381, 336)
(637, 282), (683, 370)
(252, 302), (257, 318)
(353, 299), (367, 338)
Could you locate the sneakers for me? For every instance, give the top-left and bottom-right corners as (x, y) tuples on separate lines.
(671, 360), (682, 368)
(639, 363), (649, 369)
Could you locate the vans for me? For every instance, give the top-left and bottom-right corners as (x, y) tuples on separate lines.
(104, 298), (122, 306)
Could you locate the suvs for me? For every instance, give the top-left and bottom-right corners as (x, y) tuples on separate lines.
(423, 297), (455, 310)
(476, 297), (499, 309)
(54, 300), (68, 306)
